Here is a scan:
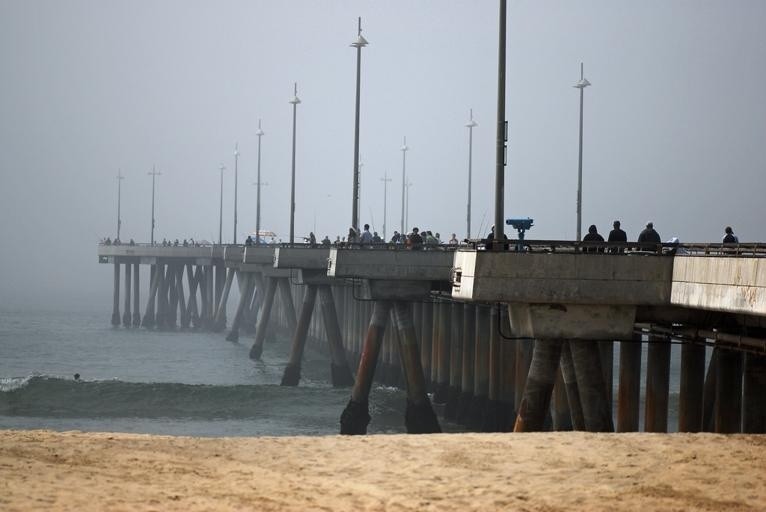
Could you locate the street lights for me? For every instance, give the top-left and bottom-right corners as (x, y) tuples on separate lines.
(400, 135), (409, 235)
(219, 163), (225, 244)
(254, 117), (264, 244)
(349, 17), (368, 231)
(574, 62), (591, 244)
(232, 142), (240, 246)
(288, 81), (302, 244)
(464, 107), (477, 241)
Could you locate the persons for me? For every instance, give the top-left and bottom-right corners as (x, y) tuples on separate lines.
(638, 221), (661, 253)
(722, 226), (739, 255)
(279, 239), (282, 244)
(484, 226), (507, 251)
(269, 238), (276, 245)
(74, 373), (82, 383)
(245, 236), (254, 244)
(103, 237), (200, 248)
(304, 223), (458, 250)
(583, 224), (606, 253)
(608, 221), (627, 252)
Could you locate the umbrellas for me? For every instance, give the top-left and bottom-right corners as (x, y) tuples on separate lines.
(258, 232), (276, 241)
